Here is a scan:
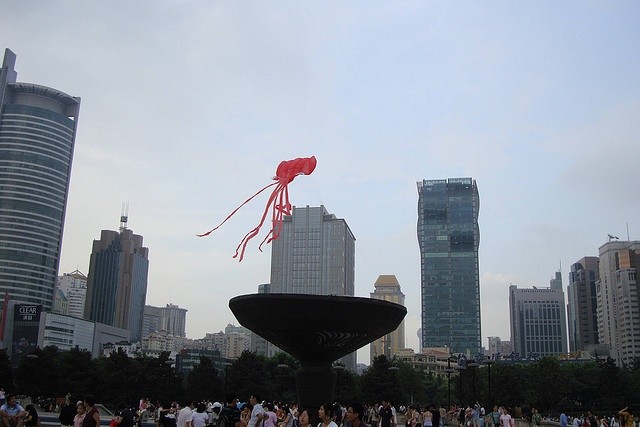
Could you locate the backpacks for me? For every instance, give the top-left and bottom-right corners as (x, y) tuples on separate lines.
(214, 406), (241, 427)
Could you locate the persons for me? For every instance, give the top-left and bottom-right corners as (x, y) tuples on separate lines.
(573, 411), (621, 427)
(57, 396), (78, 427)
(31, 395), (57, 412)
(23, 404), (42, 427)
(243, 404), (252, 427)
(269, 399), (299, 427)
(193, 404), (210, 427)
(116, 402), (134, 427)
(399, 402), (518, 427)
(82, 395), (101, 427)
(248, 394), (270, 427)
(135, 396), (177, 427)
(0, 387), (6, 409)
(618, 402), (635, 427)
(544, 414), (560, 425)
(560, 409), (570, 427)
(317, 403), (340, 427)
(0, 395), (26, 427)
(299, 409), (314, 427)
(220, 392), (242, 427)
(527, 408), (544, 427)
(342, 402), (373, 427)
(389, 401), (398, 427)
(177, 399), (194, 427)
(378, 400), (396, 427)
(73, 403), (87, 427)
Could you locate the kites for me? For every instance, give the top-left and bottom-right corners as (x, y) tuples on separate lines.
(197, 157), (319, 263)
